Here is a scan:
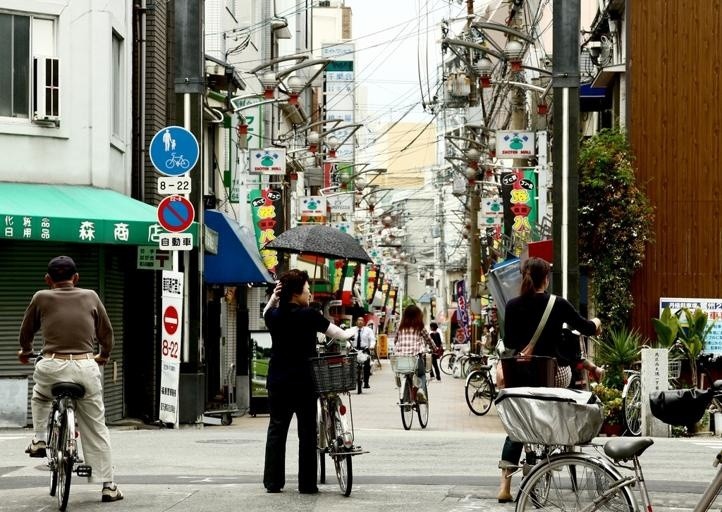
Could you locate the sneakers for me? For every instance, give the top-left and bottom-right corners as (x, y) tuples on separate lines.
(25, 440), (48, 458)
(416, 387), (426, 403)
(498, 490), (513, 503)
(101, 484), (124, 502)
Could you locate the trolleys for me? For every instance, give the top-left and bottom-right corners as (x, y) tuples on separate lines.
(203, 362), (239, 427)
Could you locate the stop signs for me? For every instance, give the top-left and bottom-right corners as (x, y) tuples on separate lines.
(164, 306), (179, 334)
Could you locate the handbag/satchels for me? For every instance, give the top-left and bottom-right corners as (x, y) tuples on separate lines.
(432, 346), (444, 357)
(496, 345), (532, 389)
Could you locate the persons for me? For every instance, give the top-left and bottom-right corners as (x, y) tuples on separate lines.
(391, 304), (437, 412)
(495, 257), (603, 504)
(427, 322), (444, 383)
(348, 316), (376, 394)
(16, 255), (125, 501)
(474, 326), (490, 382)
(259, 267), (360, 493)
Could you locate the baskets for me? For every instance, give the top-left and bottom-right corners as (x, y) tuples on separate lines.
(668, 360), (682, 380)
(310, 353), (360, 395)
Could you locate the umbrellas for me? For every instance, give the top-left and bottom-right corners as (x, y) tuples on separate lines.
(257, 220), (373, 301)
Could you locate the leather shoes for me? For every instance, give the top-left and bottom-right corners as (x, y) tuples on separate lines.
(363, 383), (370, 388)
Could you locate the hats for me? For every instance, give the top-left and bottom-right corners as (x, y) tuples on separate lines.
(47, 256), (77, 276)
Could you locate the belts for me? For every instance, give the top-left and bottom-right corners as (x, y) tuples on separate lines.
(45, 352), (95, 361)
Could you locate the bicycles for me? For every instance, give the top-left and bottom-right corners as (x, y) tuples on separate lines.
(440, 343), (500, 417)
(353, 346), (369, 393)
(310, 323), (362, 500)
(390, 345), (439, 429)
(20, 352), (113, 511)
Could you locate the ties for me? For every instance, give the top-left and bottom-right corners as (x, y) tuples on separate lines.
(357, 330), (361, 349)
